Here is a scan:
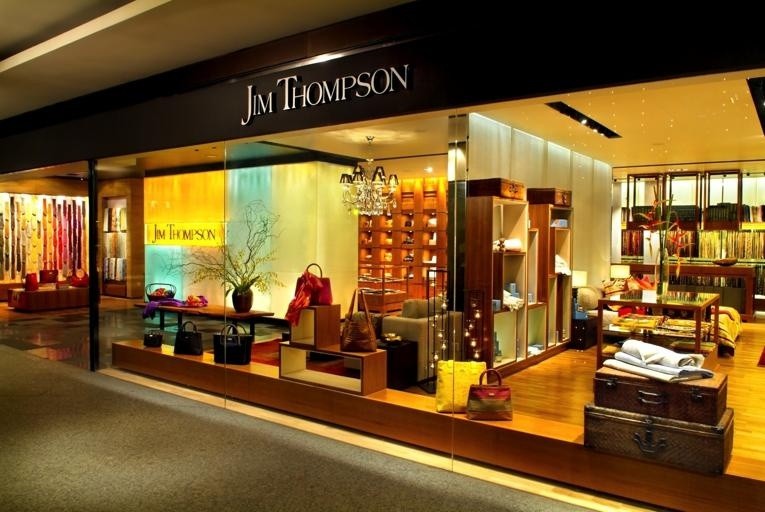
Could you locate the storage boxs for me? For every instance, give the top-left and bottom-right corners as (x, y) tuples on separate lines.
(571, 317), (596, 350)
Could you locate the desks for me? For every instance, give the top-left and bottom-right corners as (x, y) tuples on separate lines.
(597, 291), (720, 376)
(134, 301), (273, 343)
(8, 287), (99, 314)
(627, 262), (757, 324)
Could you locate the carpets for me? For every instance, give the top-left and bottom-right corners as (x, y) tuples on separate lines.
(757, 345), (765, 367)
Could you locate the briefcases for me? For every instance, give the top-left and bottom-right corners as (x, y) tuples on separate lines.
(527, 188), (571, 207)
(584, 398), (734, 474)
(593, 365), (727, 426)
(470, 178), (524, 200)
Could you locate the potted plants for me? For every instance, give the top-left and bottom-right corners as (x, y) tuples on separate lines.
(633, 185), (694, 296)
(155, 199), (288, 313)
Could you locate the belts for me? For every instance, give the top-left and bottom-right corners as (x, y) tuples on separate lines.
(0, 197), (86, 280)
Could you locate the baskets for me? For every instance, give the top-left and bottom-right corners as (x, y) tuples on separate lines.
(145, 283), (176, 302)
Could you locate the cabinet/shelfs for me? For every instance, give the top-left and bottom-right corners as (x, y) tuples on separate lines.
(468, 196), (572, 384)
(96, 181), (144, 298)
(357, 177), (449, 315)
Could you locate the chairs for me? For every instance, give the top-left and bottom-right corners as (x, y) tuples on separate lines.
(382, 311), (465, 382)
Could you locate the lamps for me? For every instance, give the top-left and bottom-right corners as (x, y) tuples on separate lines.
(572, 271), (587, 299)
(340, 136), (399, 217)
(610, 263), (630, 286)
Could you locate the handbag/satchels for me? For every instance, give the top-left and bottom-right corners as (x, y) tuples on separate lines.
(466, 369), (512, 421)
(70, 269), (89, 287)
(144, 330), (163, 347)
(295, 263), (332, 305)
(25, 273), (39, 291)
(340, 289), (378, 352)
(213, 324), (252, 364)
(40, 270), (58, 283)
(436, 360), (488, 413)
(174, 321), (202, 355)
